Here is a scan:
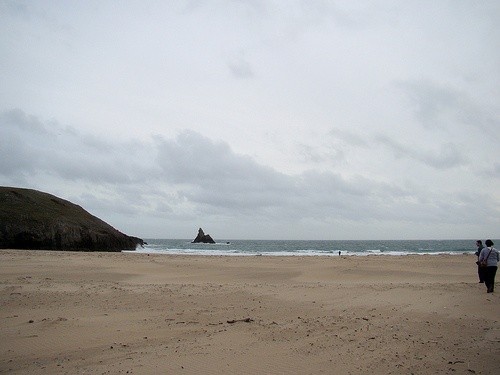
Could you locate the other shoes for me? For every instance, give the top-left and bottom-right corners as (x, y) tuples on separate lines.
(479, 281), (484, 283)
(487, 285), (494, 293)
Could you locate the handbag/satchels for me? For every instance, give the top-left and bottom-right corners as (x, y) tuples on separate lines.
(480, 259), (488, 268)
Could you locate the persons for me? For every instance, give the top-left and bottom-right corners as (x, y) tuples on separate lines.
(478, 239), (500, 294)
(473, 239), (486, 284)
